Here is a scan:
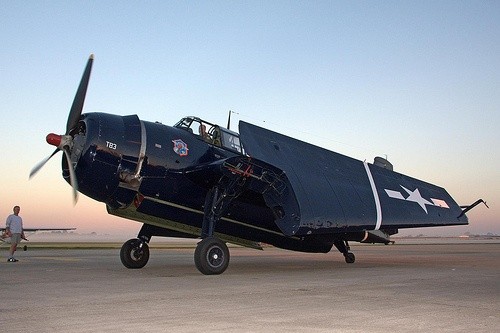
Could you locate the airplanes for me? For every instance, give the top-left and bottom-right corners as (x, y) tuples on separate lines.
(28, 52), (490, 275)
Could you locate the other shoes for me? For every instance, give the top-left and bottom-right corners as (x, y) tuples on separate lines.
(12, 257), (18, 261)
(8, 258), (16, 262)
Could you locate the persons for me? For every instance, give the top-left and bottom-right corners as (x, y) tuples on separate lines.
(199, 125), (211, 140)
(6, 205), (25, 262)
